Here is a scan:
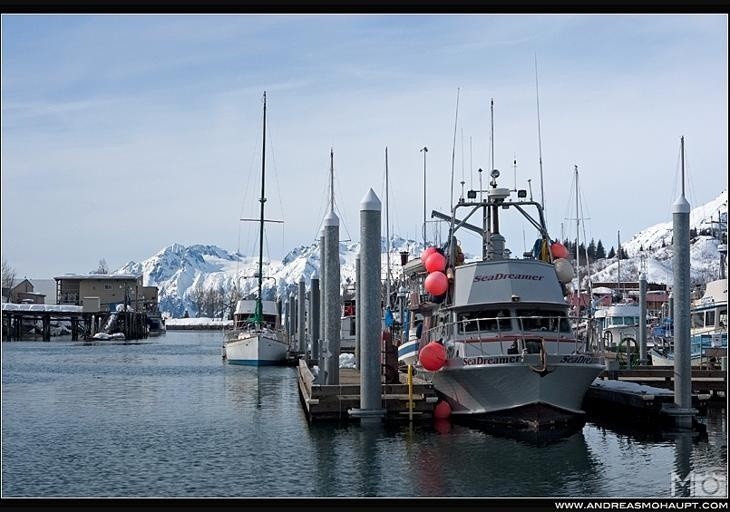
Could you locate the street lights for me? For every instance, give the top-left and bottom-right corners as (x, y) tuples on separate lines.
(670, 193), (697, 427)
(319, 219), (325, 342)
(324, 208), (340, 377)
(355, 249), (360, 372)
(356, 187), (382, 424)
(284, 273), (306, 354)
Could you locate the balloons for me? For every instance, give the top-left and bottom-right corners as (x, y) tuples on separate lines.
(550, 242), (569, 258)
(434, 400), (457, 418)
(434, 418), (451, 435)
(419, 342), (450, 372)
(423, 270), (449, 298)
(422, 246), (437, 266)
(425, 253), (448, 274)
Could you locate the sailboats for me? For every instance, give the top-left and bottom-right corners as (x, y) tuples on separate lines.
(217, 90), (296, 371)
(299, 143), (360, 354)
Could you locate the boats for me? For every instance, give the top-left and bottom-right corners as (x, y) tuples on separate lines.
(381, 48), (611, 437)
(558, 157), (727, 378)
